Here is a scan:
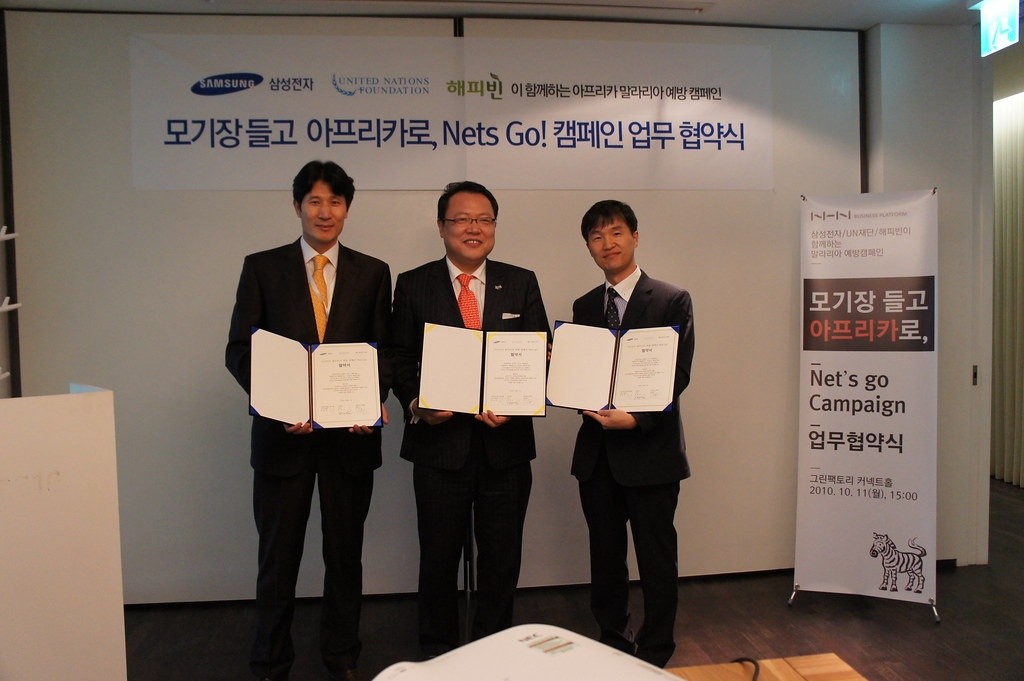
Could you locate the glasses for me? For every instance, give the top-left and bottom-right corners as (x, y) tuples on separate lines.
(443, 217), (496, 227)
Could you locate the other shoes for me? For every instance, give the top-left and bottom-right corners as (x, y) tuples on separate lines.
(330, 666), (359, 681)
(599, 625), (635, 654)
(633, 635), (675, 668)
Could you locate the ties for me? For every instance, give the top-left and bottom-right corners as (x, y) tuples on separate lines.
(456, 274), (480, 329)
(309, 256), (330, 344)
(604, 288), (621, 329)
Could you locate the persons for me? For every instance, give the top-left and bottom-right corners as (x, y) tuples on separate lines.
(546, 200), (696, 667)
(222, 158), (393, 681)
(388, 180), (552, 658)
